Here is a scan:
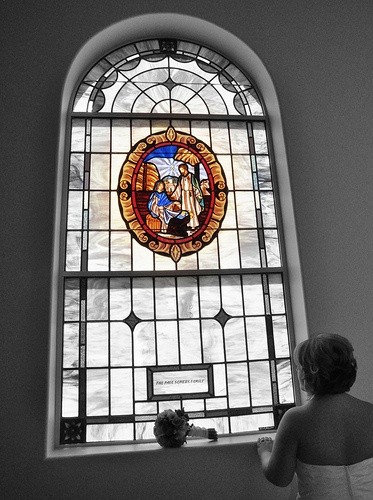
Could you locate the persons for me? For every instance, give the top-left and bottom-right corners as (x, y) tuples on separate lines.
(254, 333), (373, 500)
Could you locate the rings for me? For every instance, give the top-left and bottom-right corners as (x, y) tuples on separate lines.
(260, 439), (264, 442)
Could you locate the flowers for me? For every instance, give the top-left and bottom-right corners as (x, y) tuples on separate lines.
(152, 407), (219, 448)
